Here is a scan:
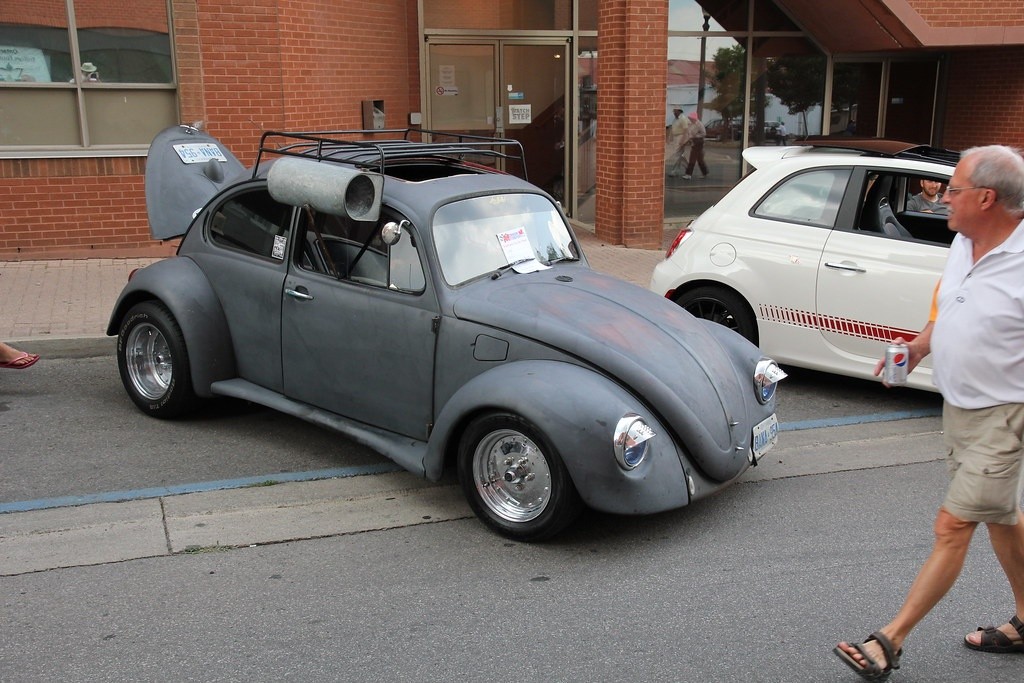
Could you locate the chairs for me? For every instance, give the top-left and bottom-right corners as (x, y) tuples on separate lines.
(860, 188), (914, 237)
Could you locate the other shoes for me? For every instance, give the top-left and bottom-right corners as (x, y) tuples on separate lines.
(699, 173), (710, 179)
(683, 174), (692, 180)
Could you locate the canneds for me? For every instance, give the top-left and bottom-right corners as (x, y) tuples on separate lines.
(885, 344), (909, 386)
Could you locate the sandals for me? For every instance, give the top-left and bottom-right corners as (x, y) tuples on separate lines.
(964, 615), (1024, 652)
(832, 632), (902, 683)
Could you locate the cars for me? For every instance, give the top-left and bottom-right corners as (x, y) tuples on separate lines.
(644, 133), (963, 400)
(106, 122), (786, 553)
(705, 119), (740, 142)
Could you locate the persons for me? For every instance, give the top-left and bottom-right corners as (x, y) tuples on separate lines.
(833, 143), (1023, 683)
(775, 121), (787, 145)
(680, 112), (710, 179)
(906, 179), (943, 212)
(69, 63), (102, 83)
(667, 106), (689, 177)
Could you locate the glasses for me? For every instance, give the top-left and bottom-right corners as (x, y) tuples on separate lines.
(947, 186), (993, 198)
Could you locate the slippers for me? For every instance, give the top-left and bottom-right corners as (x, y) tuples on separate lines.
(0, 350), (40, 368)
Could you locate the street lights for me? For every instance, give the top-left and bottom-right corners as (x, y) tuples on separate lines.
(690, 8), (712, 122)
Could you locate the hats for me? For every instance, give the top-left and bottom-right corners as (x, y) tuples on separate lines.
(688, 112), (698, 120)
(674, 106), (683, 113)
